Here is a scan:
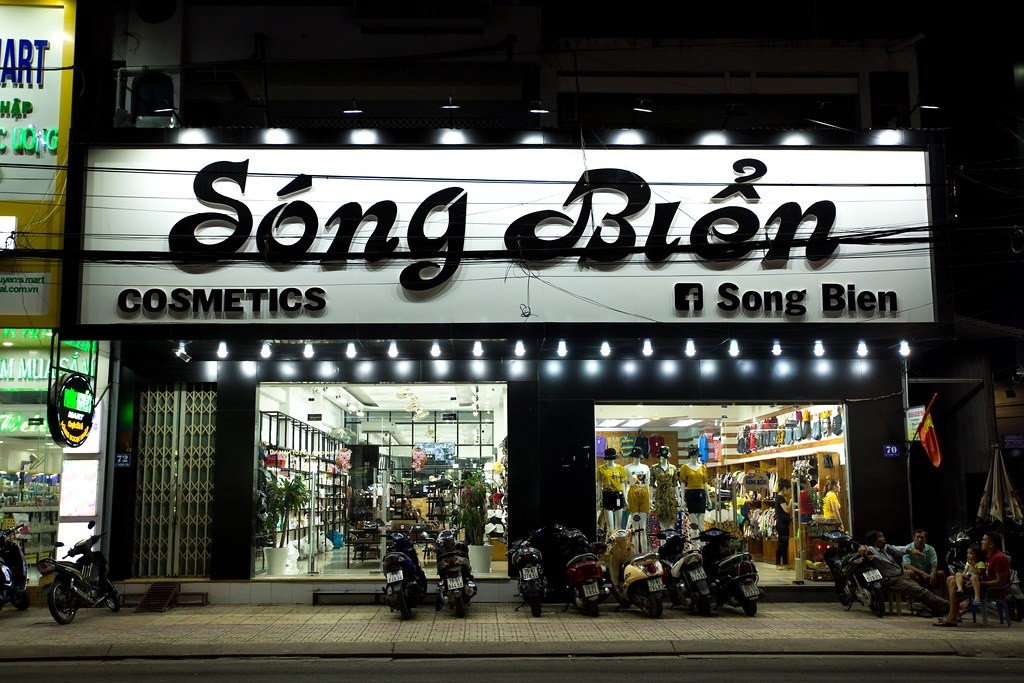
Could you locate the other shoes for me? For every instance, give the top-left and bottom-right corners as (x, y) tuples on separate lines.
(956, 590), (964, 595)
(917, 608), (933, 617)
(972, 598), (980, 605)
(776, 564), (792, 570)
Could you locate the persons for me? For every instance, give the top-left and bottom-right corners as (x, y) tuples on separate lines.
(739, 505), (754, 554)
(858, 530), (969, 617)
(931, 533), (1011, 627)
(823, 479), (845, 536)
(955, 544), (985, 606)
(650, 446), (682, 547)
(902, 528), (946, 603)
(680, 445), (712, 549)
(457, 471), (486, 543)
(596, 448), (629, 542)
(624, 446), (650, 552)
(800, 475), (816, 522)
(775, 478), (794, 570)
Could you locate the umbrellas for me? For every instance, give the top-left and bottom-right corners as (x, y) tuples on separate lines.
(975, 442), (1024, 555)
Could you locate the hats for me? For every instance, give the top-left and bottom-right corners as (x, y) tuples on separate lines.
(656, 446), (672, 459)
(603, 448), (619, 460)
(628, 447), (644, 459)
(686, 444), (703, 457)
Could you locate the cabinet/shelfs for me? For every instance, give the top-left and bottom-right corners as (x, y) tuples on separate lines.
(0, 506), (58, 565)
(254, 411), (508, 572)
(706, 452), (839, 567)
(800, 522), (842, 581)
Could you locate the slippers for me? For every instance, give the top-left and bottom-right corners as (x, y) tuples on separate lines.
(933, 616), (963, 627)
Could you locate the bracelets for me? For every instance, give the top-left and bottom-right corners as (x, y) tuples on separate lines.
(909, 548), (914, 552)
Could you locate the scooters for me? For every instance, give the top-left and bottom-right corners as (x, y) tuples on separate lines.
(645, 522), (713, 616)
(374, 517), (429, 621)
(690, 522), (767, 615)
(0, 524), (32, 614)
(596, 514), (668, 620)
(552, 522), (609, 617)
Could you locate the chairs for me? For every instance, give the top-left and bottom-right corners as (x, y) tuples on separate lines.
(885, 562), (1014, 628)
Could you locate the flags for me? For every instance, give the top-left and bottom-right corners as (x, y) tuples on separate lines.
(918, 412), (945, 469)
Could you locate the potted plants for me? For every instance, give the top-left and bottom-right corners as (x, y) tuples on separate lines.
(447, 469), (494, 573)
(260, 469), (312, 576)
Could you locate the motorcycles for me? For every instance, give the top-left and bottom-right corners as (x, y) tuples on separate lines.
(422, 508), (479, 617)
(37, 519), (120, 625)
(811, 520), (886, 618)
(946, 525), (1024, 622)
(498, 527), (551, 616)
